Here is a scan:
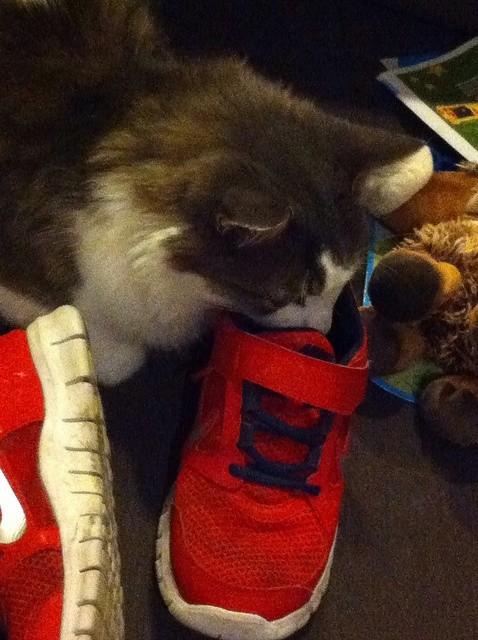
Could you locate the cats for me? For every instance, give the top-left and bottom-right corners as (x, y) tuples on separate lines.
(1, 0), (434, 386)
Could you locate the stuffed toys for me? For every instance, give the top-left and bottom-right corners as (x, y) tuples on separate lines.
(369, 213), (477, 463)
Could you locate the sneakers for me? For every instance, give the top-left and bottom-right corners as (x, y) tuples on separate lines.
(1, 304), (126, 640)
(156, 282), (368, 639)
